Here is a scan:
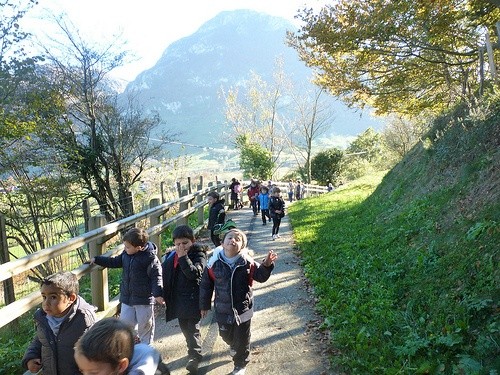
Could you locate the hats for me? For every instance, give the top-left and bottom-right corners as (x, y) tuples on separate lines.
(272, 186), (280, 193)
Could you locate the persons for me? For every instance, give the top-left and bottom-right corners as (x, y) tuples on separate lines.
(207, 191), (226, 247)
(229, 177), (343, 240)
(22, 270), (99, 375)
(74, 316), (171, 375)
(199, 229), (278, 375)
(84, 227), (165, 345)
(160, 225), (209, 373)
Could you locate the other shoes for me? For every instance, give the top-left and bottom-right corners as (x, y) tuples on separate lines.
(272, 234), (277, 241)
(262, 222), (266, 225)
(230, 346), (237, 357)
(186, 357), (199, 373)
(276, 234), (281, 238)
(269, 218), (271, 222)
(230, 365), (247, 375)
